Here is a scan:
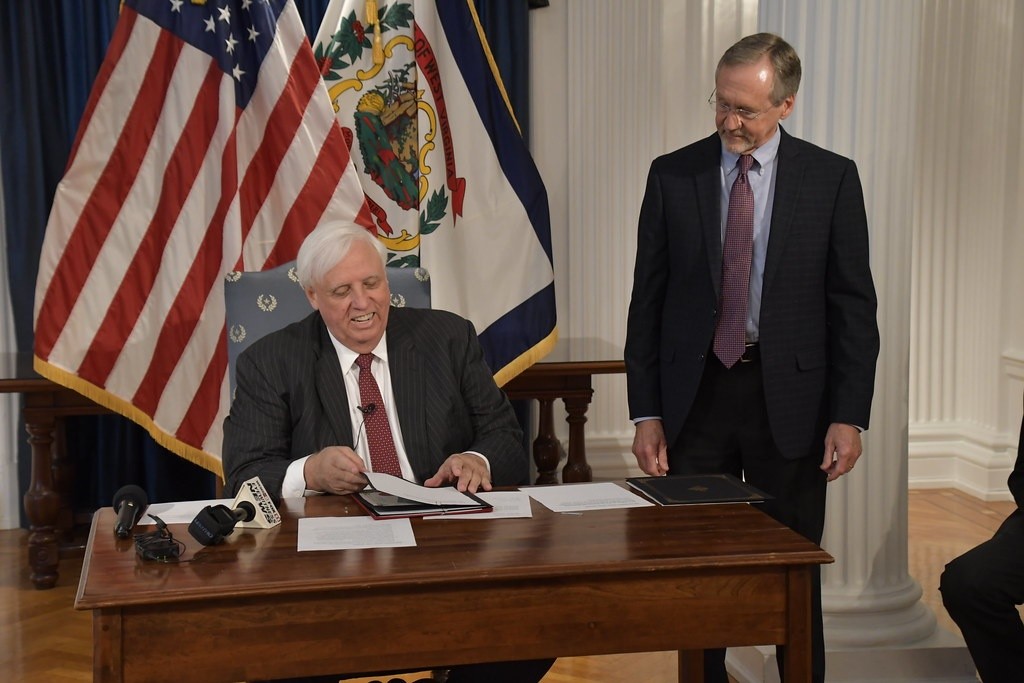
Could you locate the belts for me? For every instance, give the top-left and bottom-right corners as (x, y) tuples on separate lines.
(714, 343), (759, 363)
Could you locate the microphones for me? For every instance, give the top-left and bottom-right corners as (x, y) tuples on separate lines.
(188, 474), (283, 547)
(112, 484), (148, 539)
(368, 402), (376, 409)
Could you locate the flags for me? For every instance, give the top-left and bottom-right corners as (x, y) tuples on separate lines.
(32, 0), (559, 487)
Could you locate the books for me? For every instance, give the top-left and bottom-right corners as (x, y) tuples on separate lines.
(626, 472), (776, 506)
(352, 471), (493, 520)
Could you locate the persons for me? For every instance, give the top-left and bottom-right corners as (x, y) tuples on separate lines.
(625, 33), (880, 683)
(222, 221), (557, 683)
(938, 412), (1024, 683)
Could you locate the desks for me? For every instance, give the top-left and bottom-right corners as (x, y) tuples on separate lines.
(73, 481), (836, 683)
(0, 335), (626, 590)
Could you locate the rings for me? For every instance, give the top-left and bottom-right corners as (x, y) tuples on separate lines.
(850, 465), (854, 469)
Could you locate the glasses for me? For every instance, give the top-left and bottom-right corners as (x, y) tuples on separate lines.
(708, 88), (787, 120)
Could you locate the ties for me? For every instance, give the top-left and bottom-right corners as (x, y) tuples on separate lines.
(355, 353), (405, 479)
(713, 155), (754, 369)
